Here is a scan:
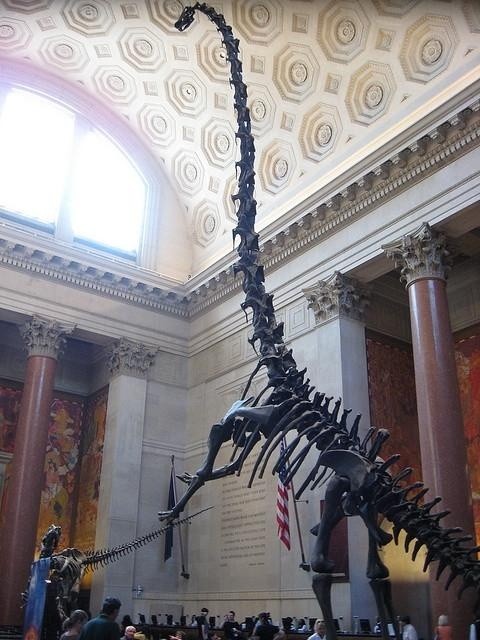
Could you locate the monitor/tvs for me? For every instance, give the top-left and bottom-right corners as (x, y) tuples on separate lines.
(140, 615), (341, 632)
(360, 619), (371, 634)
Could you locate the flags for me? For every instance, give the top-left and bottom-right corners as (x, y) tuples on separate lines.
(161, 470), (182, 566)
(275, 437), (294, 552)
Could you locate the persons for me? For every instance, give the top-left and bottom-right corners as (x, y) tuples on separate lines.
(49, 597), (144, 639)
(171, 607), (480, 639)
